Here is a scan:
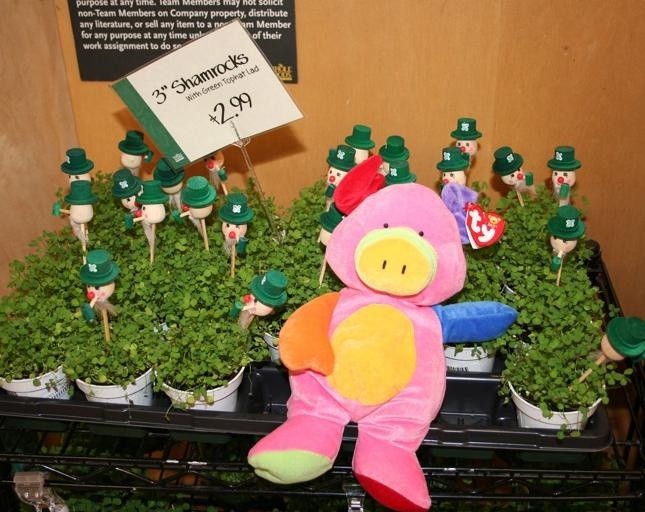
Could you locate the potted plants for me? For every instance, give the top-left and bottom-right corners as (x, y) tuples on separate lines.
(446, 289), (495, 375)
(1, 306), (74, 400)
(62, 315), (155, 406)
(260, 308), (294, 365)
(497, 325), (634, 434)
(505, 267), (589, 319)
(153, 323), (247, 412)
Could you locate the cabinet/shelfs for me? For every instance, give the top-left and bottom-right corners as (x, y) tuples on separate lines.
(0, 401), (645, 512)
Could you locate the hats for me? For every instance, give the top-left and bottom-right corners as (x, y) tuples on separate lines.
(111, 168), (143, 198)
(180, 175), (218, 208)
(546, 146), (582, 172)
(378, 135), (410, 163)
(384, 159), (417, 186)
(605, 316), (645, 357)
(60, 147), (95, 175)
(153, 157), (186, 188)
(218, 192), (255, 225)
(344, 124), (376, 149)
(118, 130), (150, 155)
(63, 180), (98, 205)
(250, 269), (289, 308)
(326, 144), (357, 172)
(491, 146), (524, 175)
(450, 117), (483, 140)
(547, 205), (585, 240)
(319, 202), (343, 233)
(436, 146), (469, 171)
(135, 179), (169, 205)
(79, 249), (120, 286)
